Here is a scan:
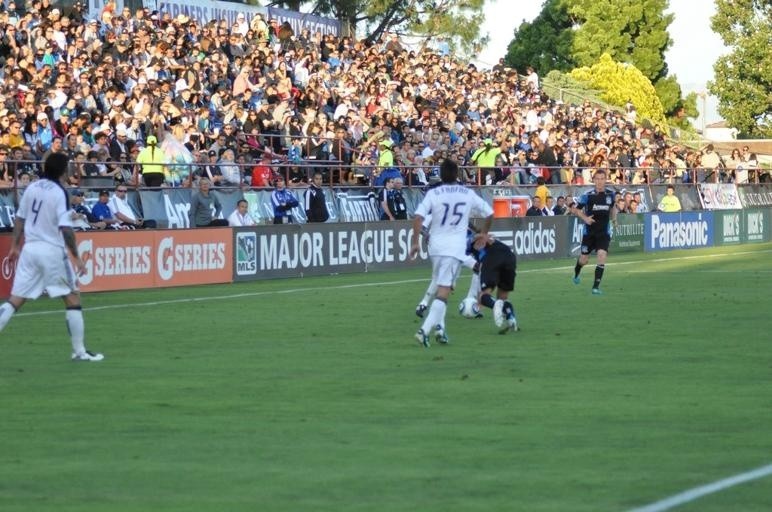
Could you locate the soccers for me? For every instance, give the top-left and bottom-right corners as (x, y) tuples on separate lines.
(460, 299), (480, 318)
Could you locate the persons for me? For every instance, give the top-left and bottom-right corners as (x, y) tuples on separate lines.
(571, 170), (619, 295)
(461, 228), (520, 334)
(408, 158), (494, 348)
(414, 221), (486, 322)
(0, 153), (105, 362)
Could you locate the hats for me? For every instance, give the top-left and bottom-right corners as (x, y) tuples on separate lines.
(60, 108), (71, 117)
(147, 134), (157, 144)
(99, 188), (110, 196)
(208, 150), (217, 157)
(113, 99), (123, 106)
(37, 112), (48, 121)
(483, 139), (493, 147)
(71, 189), (84, 195)
(379, 139), (391, 149)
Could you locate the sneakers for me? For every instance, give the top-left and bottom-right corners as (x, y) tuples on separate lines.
(493, 299), (504, 327)
(416, 304), (427, 318)
(573, 275), (581, 284)
(71, 350), (104, 362)
(414, 328), (431, 348)
(508, 317), (518, 332)
(591, 287), (601, 295)
(434, 325), (449, 345)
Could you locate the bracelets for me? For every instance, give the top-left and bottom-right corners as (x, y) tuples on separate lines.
(612, 220), (618, 223)
